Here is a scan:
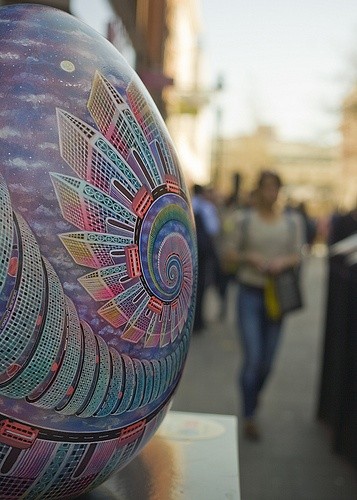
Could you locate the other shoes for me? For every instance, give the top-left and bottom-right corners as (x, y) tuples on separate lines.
(244, 425), (261, 444)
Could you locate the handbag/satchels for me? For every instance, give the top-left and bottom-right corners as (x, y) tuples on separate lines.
(264, 266), (303, 318)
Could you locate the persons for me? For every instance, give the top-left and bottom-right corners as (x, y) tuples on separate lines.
(219, 167), (310, 439)
(187, 172), (317, 336)
